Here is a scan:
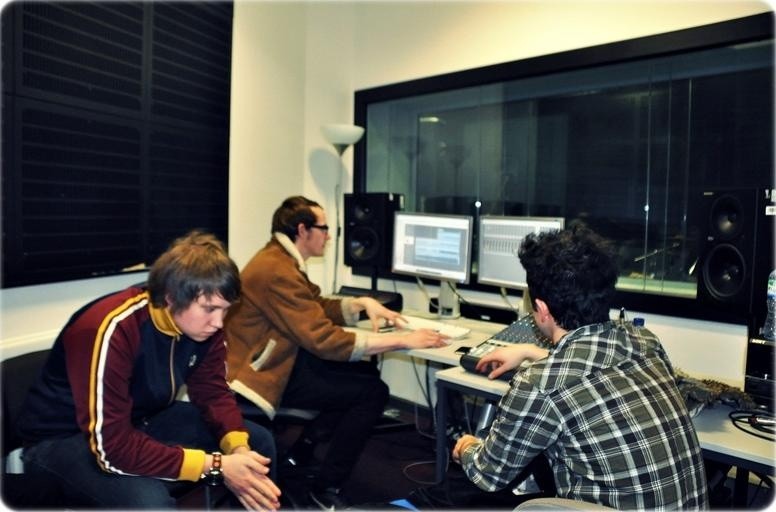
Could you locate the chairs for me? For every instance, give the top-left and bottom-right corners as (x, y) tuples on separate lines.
(512, 498), (620, 511)
(237, 402), (341, 508)
(3, 348), (217, 508)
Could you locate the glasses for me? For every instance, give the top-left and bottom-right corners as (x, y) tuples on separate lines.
(311, 224), (329, 233)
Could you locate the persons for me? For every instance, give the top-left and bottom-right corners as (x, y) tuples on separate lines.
(453, 228), (709, 510)
(225, 195), (448, 509)
(19, 228), (281, 512)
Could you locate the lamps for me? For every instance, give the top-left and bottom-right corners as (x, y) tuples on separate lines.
(319, 123), (365, 295)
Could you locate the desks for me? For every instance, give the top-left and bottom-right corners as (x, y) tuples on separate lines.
(435, 366), (775, 511)
(348, 308), (509, 428)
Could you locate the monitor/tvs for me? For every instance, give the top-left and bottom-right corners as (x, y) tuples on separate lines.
(392, 211), (474, 319)
(478, 216), (565, 320)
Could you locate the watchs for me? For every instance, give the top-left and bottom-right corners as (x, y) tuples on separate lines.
(205, 450), (224, 485)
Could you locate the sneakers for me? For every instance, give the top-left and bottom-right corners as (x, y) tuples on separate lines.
(310, 485), (352, 511)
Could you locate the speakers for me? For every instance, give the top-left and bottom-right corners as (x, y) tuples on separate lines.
(696, 187), (776, 314)
(344, 192), (404, 266)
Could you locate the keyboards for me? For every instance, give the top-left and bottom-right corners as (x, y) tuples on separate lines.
(389, 315), (471, 339)
